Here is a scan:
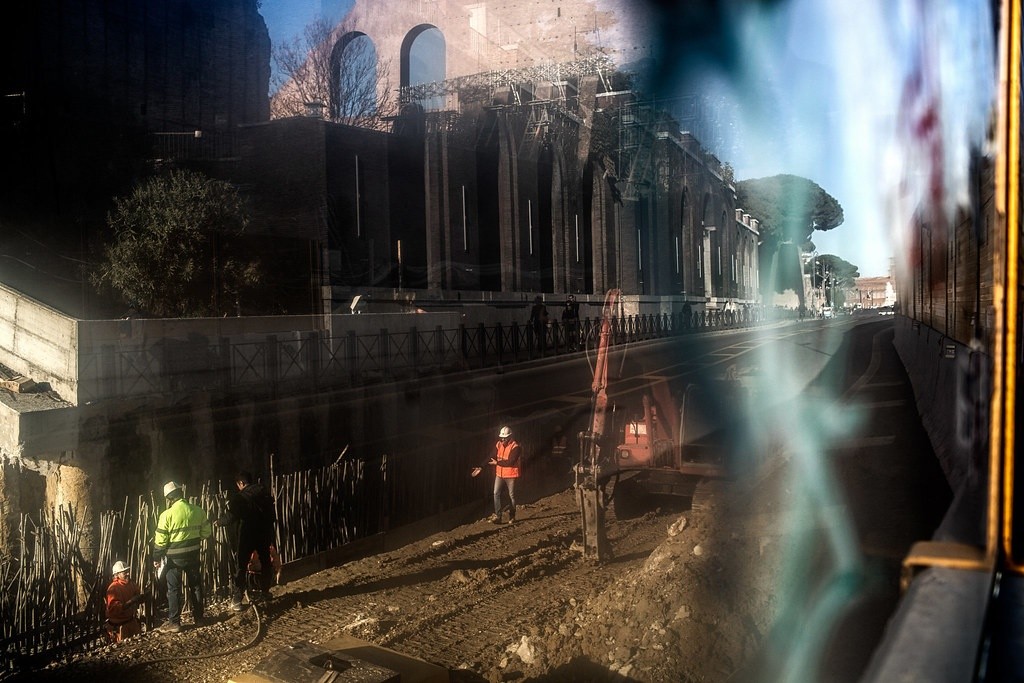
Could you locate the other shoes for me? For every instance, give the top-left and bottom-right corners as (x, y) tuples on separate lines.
(488, 514), (502, 524)
(195, 618), (207, 626)
(509, 518), (514, 523)
(160, 623), (180, 633)
(228, 602), (241, 611)
(261, 592), (272, 600)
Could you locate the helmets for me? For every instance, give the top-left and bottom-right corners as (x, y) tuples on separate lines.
(164, 481), (182, 497)
(112, 561), (130, 575)
(498, 426), (513, 437)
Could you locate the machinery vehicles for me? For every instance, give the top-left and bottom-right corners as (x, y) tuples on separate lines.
(575, 288), (763, 566)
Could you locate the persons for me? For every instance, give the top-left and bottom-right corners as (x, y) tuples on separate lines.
(743, 301), (750, 309)
(472, 426), (521, 524)
(725, 299), (737, 319)
(542, 425), (573, 475)
(799, 301), (805, 320)
(153, 481), (211, 633)
(215, 471), (276, 611)
(530, 296), (551, 357)
(562, 295), (580, 349)
(682, 301), (693, 328)
(106, 560), (148, 642)
